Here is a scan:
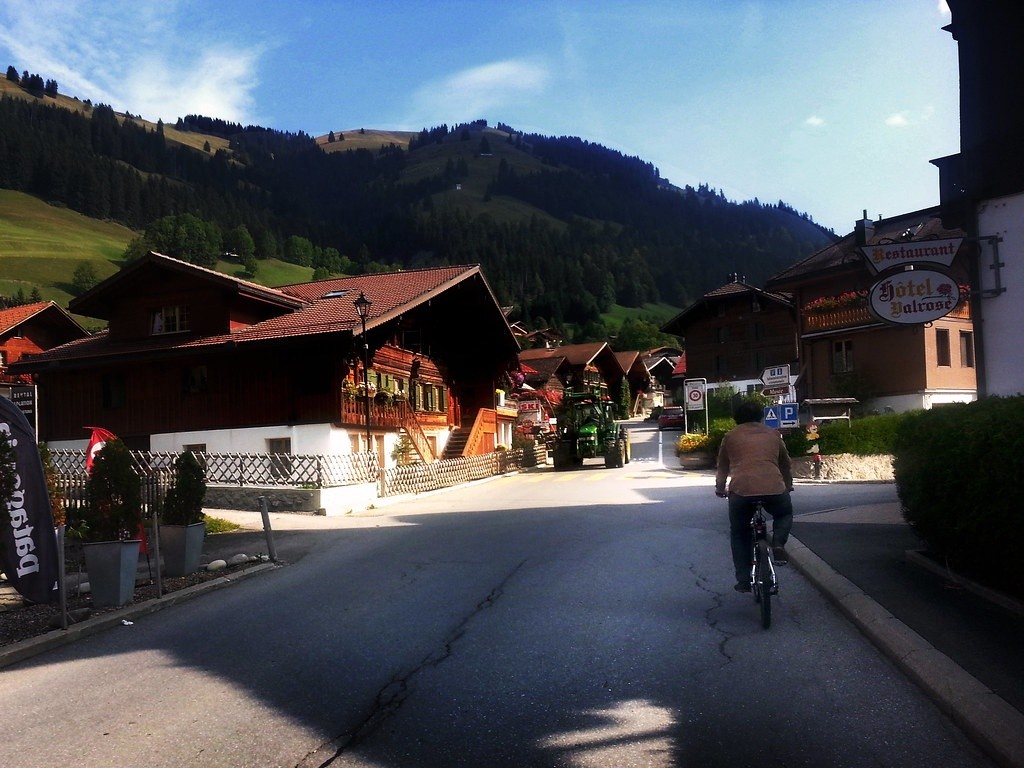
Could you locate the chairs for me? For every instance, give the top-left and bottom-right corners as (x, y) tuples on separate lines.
(585, 415), (597, 424)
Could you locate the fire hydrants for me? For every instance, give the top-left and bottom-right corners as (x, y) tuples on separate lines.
(812, 455), (824, 480)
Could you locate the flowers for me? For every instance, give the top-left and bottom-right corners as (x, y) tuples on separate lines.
(356, 381), (376, 393)
(800, 290), (869, 317)
(673, 432), (715, 457)
(959, 284), (971, 302)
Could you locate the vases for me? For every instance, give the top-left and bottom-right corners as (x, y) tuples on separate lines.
(677, 451), (713, 470)
(360, 391), (375, 398)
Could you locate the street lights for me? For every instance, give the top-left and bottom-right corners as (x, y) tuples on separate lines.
(353, 290), (374, 479)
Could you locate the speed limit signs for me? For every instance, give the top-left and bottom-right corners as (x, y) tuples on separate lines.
(689, 389), (701, 401)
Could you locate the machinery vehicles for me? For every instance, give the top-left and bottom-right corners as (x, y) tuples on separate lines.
(552, 390), (631, 471)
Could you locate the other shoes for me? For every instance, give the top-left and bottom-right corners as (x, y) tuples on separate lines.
(733, 582), (751, 592)
(772, 540), (790, 563)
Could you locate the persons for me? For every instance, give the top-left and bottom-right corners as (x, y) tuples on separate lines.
(715, 399), (795, 593)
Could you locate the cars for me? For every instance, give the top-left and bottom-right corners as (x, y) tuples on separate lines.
(658, 406), (685, 430)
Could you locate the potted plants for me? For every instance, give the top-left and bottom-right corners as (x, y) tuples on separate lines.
(79, 437), (144, 609)
(37, 442), (66, 589)
(157, 449), (206, 578)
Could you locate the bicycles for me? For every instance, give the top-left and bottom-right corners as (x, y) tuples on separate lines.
(715, 487), (794, 629)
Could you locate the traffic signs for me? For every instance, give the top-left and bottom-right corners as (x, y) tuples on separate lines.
(758, 364), (790, 386)
(759, 385), (790, 398)
